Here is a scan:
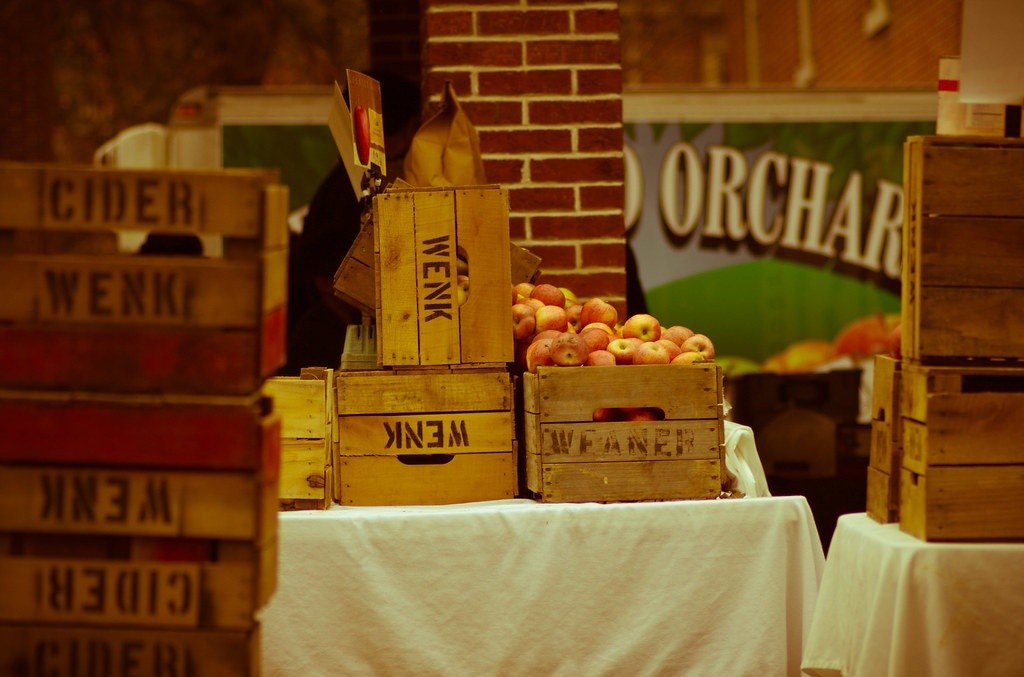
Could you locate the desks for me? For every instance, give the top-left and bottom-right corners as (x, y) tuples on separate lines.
(798, 510), (1024, 677)
(259, 494), (828, 676)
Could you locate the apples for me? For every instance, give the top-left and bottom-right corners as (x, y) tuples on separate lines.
(456, 274), (715, 421)
(716, 310), (901, 373)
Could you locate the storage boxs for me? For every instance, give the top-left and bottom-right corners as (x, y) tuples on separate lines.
(268, 185), (728, 508)
(728, 368), (861, 480)
(0, 162), (288, 677)
(866, 135), (1024, 543)
